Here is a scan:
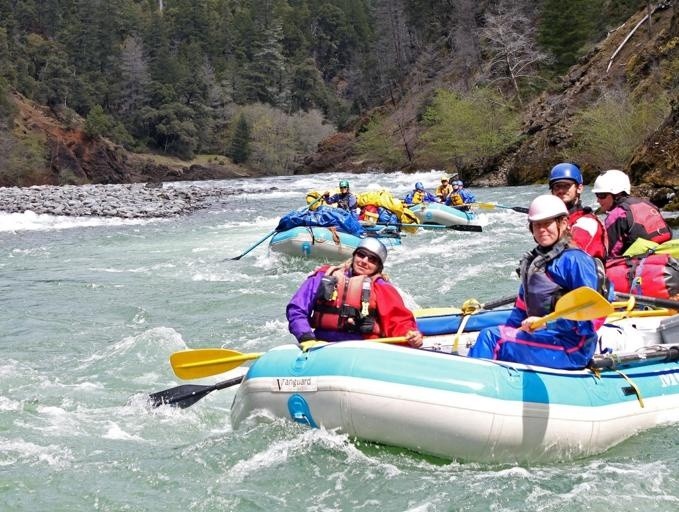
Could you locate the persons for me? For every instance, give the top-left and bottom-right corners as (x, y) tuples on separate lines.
(463, 193), (610, 370)
(591, 169), (675, 264)
(547, 160), (610, 265)
(281, 236), (425, 351)
(322, 178), (357, 214)
(434, 176), (453, 204)
(403, 182), (441, 205)
(457, 180), (476, 204)
(442, 181), (468, 212)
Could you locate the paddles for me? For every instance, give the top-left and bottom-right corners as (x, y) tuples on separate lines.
(146, 337), (412, 413)
(223, 192), (326, 262)
(517, 286), (617, 333)
(377, 222), (482, 233)
(475, 200), (530, 213)
(410, 294), (516, 318)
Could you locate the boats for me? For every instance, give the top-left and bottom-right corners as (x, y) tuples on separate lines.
(407, 201), (478, 228)
(267, 226), (404, 265)
(228, 307), (679, 478)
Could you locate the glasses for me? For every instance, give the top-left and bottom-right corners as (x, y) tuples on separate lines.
(596, 193), (613, 197)
(353, 250), (380, 264)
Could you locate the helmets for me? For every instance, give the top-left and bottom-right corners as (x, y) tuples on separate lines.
(457, 180), (464, 186)
(414, 182), (425, 191)
(591, 167), (632, 194)
(527, 193), (570, 222)
(338, 180), (350, 188)
(452, 181), (458, 186)
(441, 176), (449, 183)
(354, 234), (389, 265)
(548, 162), (584, 190)
